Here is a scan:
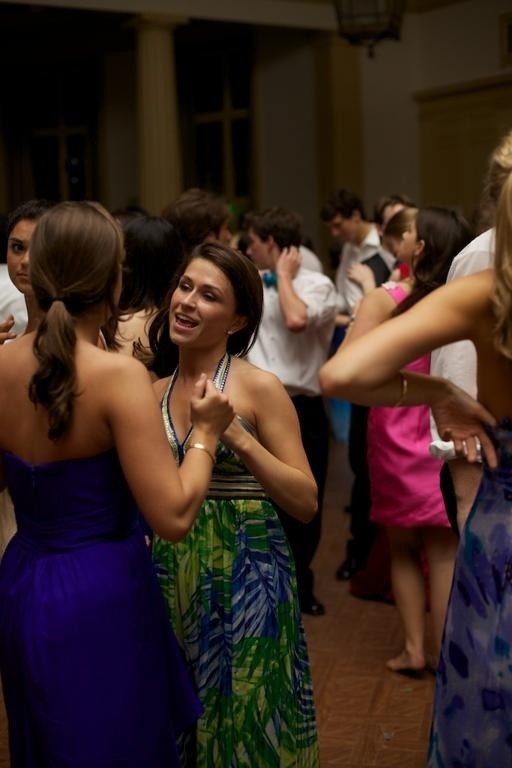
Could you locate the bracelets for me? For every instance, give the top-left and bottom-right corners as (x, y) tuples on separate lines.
(393, 372), (408, 408)
(185, 443), (217, 465)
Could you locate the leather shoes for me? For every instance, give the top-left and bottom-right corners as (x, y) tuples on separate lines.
(300, 595), (325, 615)
(336, 557), (354, 581)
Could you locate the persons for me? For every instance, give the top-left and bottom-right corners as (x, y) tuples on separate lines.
(0, 198), (237, 767)
(145, 237), (323, 768)
(2, 191), (511, 676)
(316, 130), (512, 767)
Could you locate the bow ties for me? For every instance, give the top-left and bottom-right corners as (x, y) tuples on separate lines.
(263, 271), (278, 288)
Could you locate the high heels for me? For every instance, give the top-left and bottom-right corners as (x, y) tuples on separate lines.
(384, 651), (427, 679)
